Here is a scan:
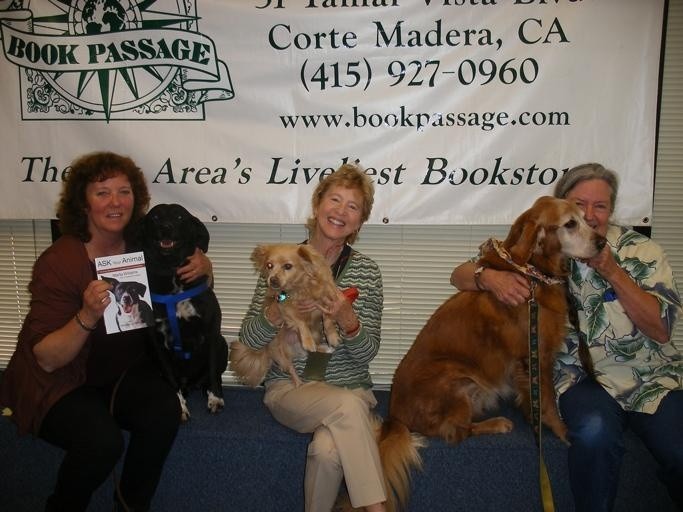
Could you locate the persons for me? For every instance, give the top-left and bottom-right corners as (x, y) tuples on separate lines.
(237, 162), (390, 512)
(1, 151), (215, 510)
(450, 162), (683, 512)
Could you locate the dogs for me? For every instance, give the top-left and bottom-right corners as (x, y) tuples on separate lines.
(332, 194), (607, 512)
(228, 242), (345, 392)
(101, 275), (157, 333)
(122, 204), (228, 423)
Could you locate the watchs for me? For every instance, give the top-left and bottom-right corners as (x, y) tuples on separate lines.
(474, 266), (487, 291)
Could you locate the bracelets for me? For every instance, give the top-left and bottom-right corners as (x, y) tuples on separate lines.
(346, 321), (360, 334)
(75, 313), (97, 331)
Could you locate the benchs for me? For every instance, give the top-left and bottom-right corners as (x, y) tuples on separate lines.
(0, 385), (679, 512)
